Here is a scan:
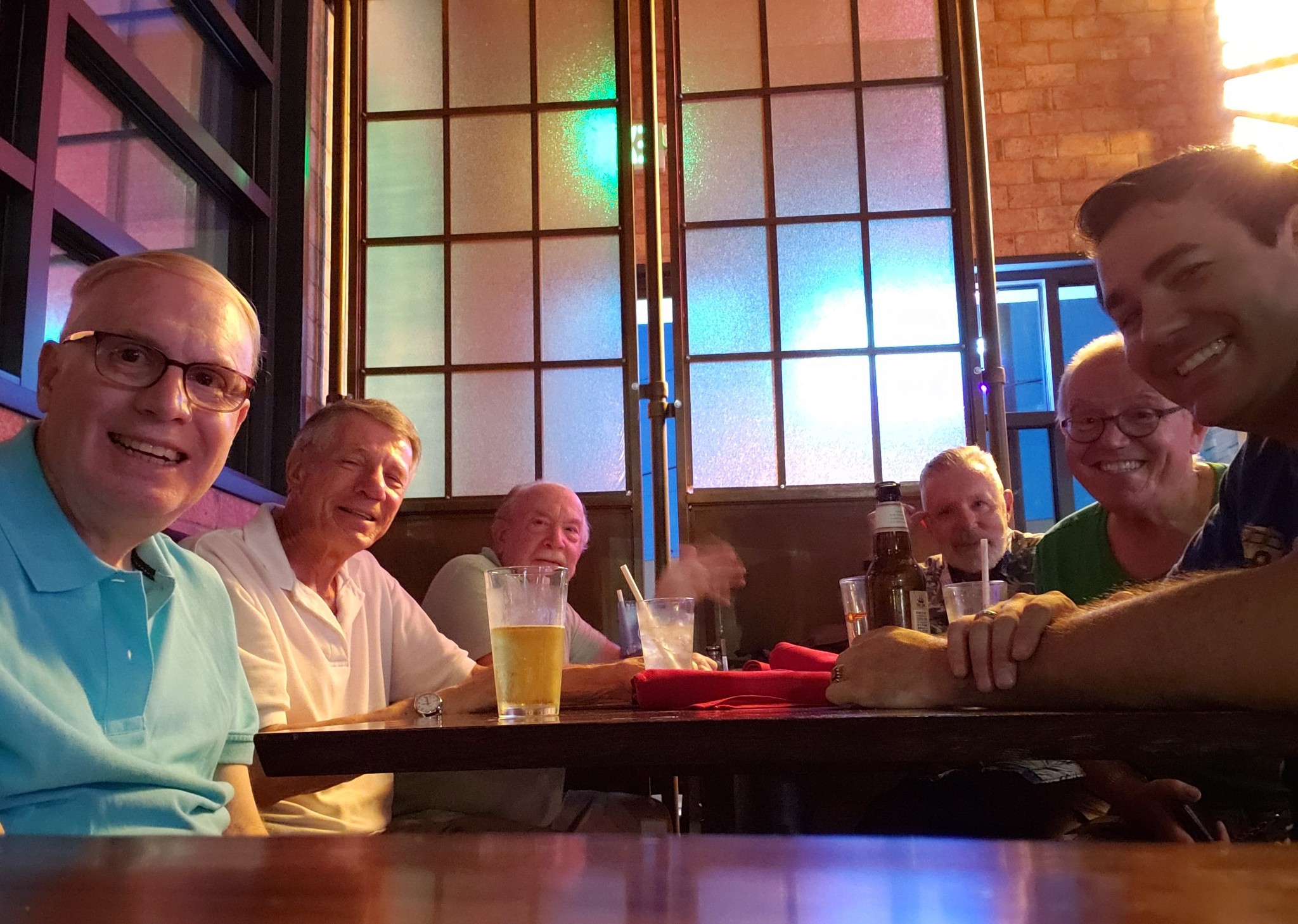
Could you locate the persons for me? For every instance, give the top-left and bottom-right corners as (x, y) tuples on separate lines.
(194, 398), (746, 836)
(825, 148), (1298, 710)
(0, 251), (272, 835)
(917, 445), (1048, 634)
(1031, 330), (1298, 843)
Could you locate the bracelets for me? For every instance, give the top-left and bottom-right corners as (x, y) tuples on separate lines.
(1103, 768), (1140, 802)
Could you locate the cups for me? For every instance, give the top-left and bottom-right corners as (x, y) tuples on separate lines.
(839, 574), (868, 647)
(635, 597), (695, 670)
(941, 580), (1008, 623)
(483, 564), (570, 725)
(617, 600), (643, 660)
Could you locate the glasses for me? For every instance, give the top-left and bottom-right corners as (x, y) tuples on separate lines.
(1061, 406), (1184, 444)
(62, 331), (258, 412)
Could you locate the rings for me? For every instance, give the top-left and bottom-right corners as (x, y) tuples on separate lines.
(831, 664), (844, 683)
(974, 609), (997, 619)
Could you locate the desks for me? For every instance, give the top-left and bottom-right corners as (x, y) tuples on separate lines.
(253, 661), (1298, 844)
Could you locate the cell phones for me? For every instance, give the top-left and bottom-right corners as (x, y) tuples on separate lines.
(1168, 801), (1214, 845)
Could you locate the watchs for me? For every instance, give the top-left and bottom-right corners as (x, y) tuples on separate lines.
(413, 691), (445, 723)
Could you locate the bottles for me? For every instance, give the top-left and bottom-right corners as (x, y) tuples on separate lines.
(704, 646), (723, 671)
(865, 480), (931, 636)
